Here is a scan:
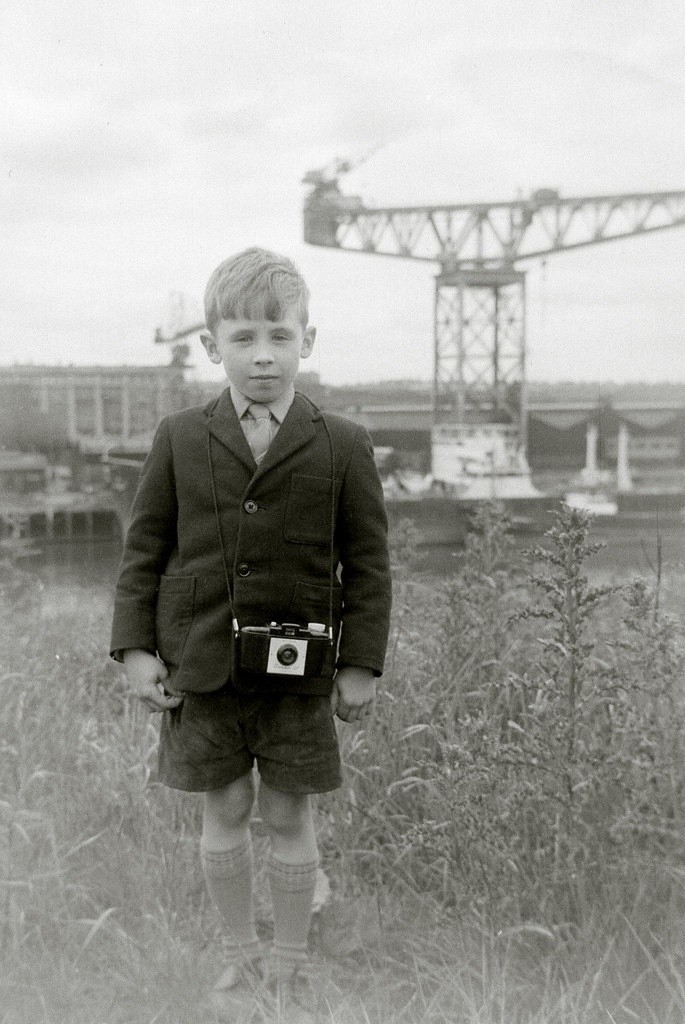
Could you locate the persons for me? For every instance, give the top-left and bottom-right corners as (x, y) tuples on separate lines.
(109, 246), (392, 1024)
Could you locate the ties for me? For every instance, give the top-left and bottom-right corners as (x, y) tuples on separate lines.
(248, 403), (273, 466)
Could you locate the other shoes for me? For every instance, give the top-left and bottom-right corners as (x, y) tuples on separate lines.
(214, 958), (262, 993)
(279, 973), (318, 1013)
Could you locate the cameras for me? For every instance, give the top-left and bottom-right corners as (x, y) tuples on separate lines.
(233, 622), (337, 698)
